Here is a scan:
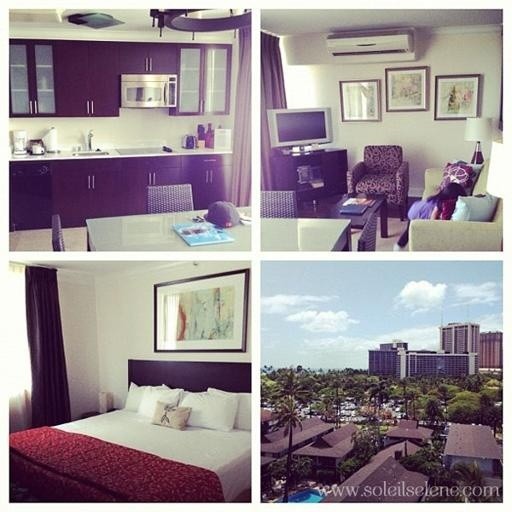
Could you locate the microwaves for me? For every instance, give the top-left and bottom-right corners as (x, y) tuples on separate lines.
(120, 73), (178, 109)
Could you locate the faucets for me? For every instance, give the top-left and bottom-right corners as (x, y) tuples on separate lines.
(87, 128), (97, 151)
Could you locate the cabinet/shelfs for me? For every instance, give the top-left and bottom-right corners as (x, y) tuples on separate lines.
(181, 151), (235, 212)
(118, 41), (181, 78)
(54, 39), (121, 119)
(166, 43), (232, 117)
(123, 153), (184, 217)
(50, 156), (126, 229)
(9, 37), (57, 119)
(9, 159), (52, 232)
(268, 145), (348, 211)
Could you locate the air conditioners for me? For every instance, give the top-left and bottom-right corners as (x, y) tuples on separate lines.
(323, 27), (418, 63)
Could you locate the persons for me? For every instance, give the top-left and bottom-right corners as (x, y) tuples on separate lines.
(393, 182), (468, 251)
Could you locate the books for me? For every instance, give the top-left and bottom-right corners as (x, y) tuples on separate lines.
(173, 221), (233, 247)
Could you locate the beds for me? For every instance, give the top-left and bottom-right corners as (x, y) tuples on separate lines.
(322, 189), (389, 240)
(10, 358), (253, 502)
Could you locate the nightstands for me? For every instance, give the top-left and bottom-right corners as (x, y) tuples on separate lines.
(81, 407), (121, 420)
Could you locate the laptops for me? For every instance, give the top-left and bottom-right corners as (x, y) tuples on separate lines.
(339, 204), (368, 216)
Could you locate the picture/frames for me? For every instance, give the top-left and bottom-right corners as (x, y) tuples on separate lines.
(384, 66), (431, 112)
(432, 74), (481, 121)
(150, 267), (251, 353)
(337, 78), (382, 124)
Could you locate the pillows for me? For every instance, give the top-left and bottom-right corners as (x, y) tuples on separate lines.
(125, 376), (251, 433)
(446, 192), (501, 223)
(435, 160), (484, 199)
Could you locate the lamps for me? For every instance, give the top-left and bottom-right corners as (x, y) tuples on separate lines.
(463, 115), (494, 165)
(147, 9), (252, 42)
(485, 137), (503, 252)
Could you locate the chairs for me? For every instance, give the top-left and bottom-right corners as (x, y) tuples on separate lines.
(344, 144), (410, 222)
(144, 179), (195, 215)
(356, 210), (377, 252)
(50, 212), (67, 252)
(258, 189), (299, 220)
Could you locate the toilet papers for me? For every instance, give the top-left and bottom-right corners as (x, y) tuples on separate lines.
(42, 125), (59, 154)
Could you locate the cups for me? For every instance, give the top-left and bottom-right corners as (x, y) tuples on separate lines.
(32, 145), (45, 154)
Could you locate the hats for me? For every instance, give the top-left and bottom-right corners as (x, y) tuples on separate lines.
(204, 200), (240, 229)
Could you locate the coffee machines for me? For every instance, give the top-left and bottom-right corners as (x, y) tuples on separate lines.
(13, 130), (29, 154)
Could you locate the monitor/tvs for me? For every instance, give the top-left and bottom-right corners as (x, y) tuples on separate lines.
(266, 107), (333, 154)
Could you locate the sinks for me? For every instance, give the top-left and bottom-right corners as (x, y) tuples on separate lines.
(71, 151), (112, 158)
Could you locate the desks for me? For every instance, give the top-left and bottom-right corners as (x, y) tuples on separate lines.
(258, 216), (353, 253)
(84, 203), (253, 252)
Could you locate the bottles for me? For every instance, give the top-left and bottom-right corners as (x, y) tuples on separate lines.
(197, 122), (215, 150)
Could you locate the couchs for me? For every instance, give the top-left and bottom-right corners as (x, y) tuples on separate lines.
(405, 157), (503, 254)
